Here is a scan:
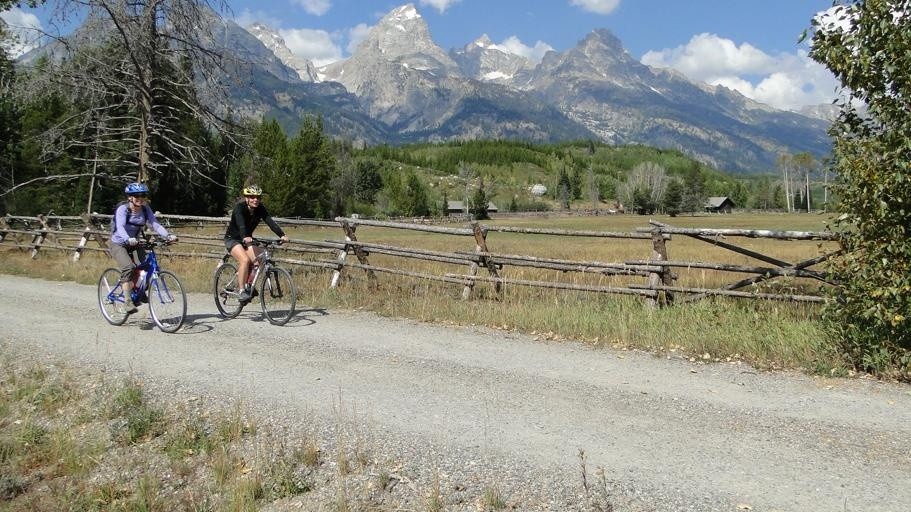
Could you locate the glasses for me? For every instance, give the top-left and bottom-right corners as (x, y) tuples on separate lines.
(129, 194), (147, 198)
(250, 196), (262, 200)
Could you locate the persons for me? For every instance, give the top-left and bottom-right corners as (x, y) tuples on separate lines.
(223, 183), (290, 303)
(109, 182), (178, 315)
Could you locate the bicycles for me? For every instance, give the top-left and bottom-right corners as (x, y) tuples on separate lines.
(213, 240), (297, 326)
(98, 240), (187, 333)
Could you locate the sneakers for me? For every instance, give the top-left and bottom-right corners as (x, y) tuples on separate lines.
(245, 283), (259, 296)
(238, 290), (251, 302)
(124, 298), (138, 314)
(141, 293), (149, 303)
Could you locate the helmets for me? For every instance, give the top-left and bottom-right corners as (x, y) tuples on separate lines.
(243, 184), (263, 196)
(125, 182), (149, 194)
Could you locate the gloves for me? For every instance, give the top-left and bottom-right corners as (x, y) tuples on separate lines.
(168, 234), (177, 241)
(128, 237), (139, 247)
